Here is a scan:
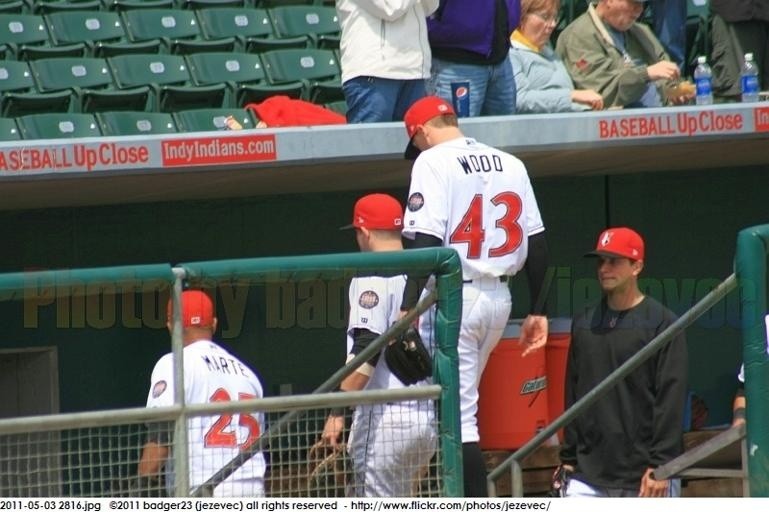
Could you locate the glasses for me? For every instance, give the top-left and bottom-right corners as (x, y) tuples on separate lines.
(533, 10), (560, 25)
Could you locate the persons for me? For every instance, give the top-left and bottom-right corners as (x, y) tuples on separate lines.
(127, 289), (268, 497)
(552, 228), (687, 497)
(320, 194), (439, 498)
(389, 97), (555, 497)
(731, 314), (769, 428)
(336, 0), (765, 124)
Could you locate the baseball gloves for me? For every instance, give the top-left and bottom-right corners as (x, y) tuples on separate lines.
(386, 320), (434, 386)
(309, 441), (352, 495)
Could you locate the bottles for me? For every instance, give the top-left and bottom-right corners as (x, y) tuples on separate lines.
(694, 56), (712, 104)
(740, 52), (760, 103)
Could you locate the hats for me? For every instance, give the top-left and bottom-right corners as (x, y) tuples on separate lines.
(340, 194), (403, 230)
(404, 97), (455, 159)
(166, 291), (214, 327)
(583, 228), (644, 263)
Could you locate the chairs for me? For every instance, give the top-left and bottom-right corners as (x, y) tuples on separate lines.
(685, 0), (713, 65)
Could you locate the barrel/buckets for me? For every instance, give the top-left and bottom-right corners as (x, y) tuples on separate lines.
(477, 314), (574, 452)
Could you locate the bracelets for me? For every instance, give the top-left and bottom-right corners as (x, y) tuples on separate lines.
(329, 390), (348, 416)
